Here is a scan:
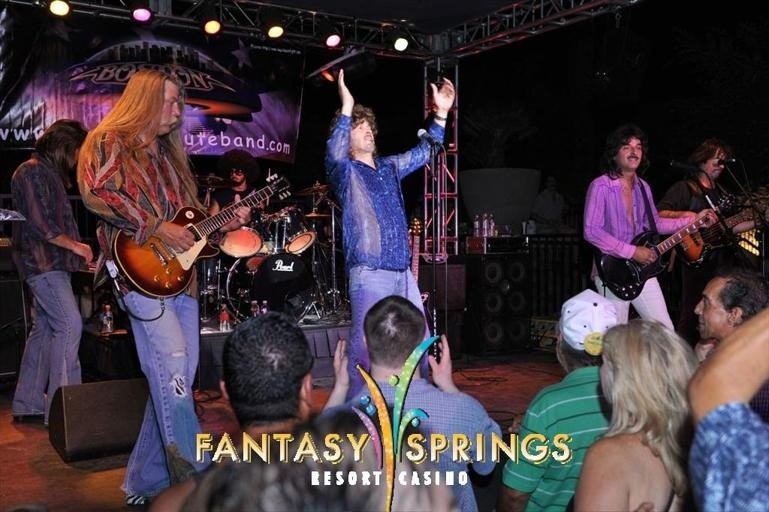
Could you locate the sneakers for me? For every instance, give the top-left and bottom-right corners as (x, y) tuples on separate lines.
(125, 493), (151, 506)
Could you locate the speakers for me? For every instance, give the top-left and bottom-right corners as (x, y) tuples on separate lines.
(460, 253), (533, 357)
(48, 377), (150, 463)
(0, 275), (33, 391)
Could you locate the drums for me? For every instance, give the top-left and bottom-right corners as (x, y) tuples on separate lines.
(218, 205), (264, 258)
(225, 241), (315, 324)
(262, 204), (317, 255)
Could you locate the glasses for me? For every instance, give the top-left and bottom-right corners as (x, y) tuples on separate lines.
(229, 168), (242, 176)
(712, 158), (721, 167)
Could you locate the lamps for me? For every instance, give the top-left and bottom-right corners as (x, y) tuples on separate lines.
(49, 0), (72, 17)
(261, 6), (284, 41)
(131, 0), (154, 24)
(201, 3), (222, 35)
(390, 28), (410, 52)
(317, 17), (342, 48)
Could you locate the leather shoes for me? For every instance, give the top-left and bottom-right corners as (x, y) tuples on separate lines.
(13, 415), (44, 423)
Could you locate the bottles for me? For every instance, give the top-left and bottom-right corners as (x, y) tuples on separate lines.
(488, 211), (496, 238)
(219, 303), (231, 331)
(102, 305), (113, 332)
(260, 300), (269, 316)
(250, 300), (260, 317)
(473, 213), (481, 237)
(479, 212), (488, 237)
(98, 303), (106, 329)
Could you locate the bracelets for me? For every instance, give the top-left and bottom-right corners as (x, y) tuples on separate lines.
(435, 115), (447, 121)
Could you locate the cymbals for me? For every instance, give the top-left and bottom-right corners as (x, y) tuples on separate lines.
(198, 175), (235, 189)
(298, 185), (330, 195)
(304, 214), (331, 217)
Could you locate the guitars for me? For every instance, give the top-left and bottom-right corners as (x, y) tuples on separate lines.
(600, 192), (737, 301)
(411, 217), (433, 333)
(674, 207), (759, 268)
(112, 173), (291, 299)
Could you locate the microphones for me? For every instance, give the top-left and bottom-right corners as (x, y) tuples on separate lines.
(215, 176), (231, 184)
(670, 160), (704, 172)
(712, 158), (736, 166)
(417, 128), (443, 147)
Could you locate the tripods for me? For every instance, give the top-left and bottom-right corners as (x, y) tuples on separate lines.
(306, 193), (347, 318)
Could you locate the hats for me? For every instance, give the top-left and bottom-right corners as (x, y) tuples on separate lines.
(559, 289), (617, 351)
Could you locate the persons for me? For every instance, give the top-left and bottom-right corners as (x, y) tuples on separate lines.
(684, 306), (769, 511)
(692, 269), (767, 423)
(207, 148), (268, 325)
(530, 174), (569, 231)
(326, 69), (459, 407)
(574, 319), (697, 511)
(322, 295), (502, 510)
(76, 68), (252, 506)
(653, 137), (767, 317)
(501, 289), (617, 511)
(9, 120), (99, 428)
(582, 125), (720, 330)
(148, 310), (388, 511)
